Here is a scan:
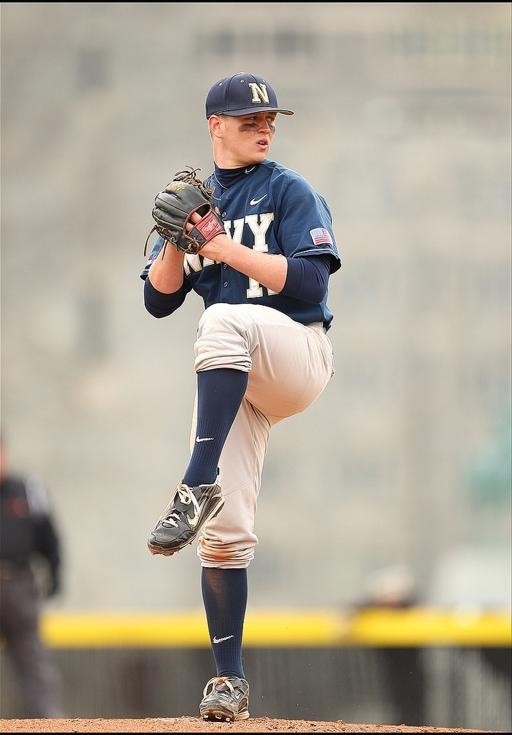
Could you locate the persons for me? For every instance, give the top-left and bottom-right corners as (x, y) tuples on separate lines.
(357, 562), (430, 724)
(1, 434), (65, 718)
(139, 73), (342, 722)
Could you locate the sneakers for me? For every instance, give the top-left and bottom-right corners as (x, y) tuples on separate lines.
(198, 676), (251, 721)
(147, 477), (226, 557)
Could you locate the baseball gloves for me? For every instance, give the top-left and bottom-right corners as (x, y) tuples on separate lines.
(143, 167), (227, 260)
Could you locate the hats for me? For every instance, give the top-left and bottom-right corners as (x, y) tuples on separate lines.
(206, 72), (294, 120)
